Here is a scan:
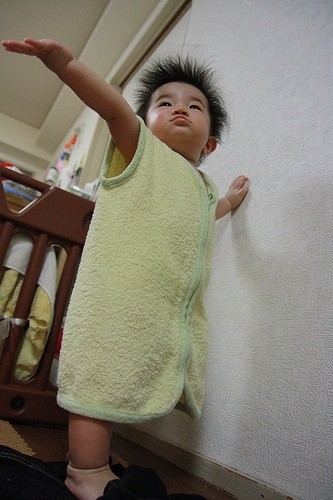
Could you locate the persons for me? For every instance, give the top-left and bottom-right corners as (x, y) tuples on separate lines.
(2, 38), (249, 500)
